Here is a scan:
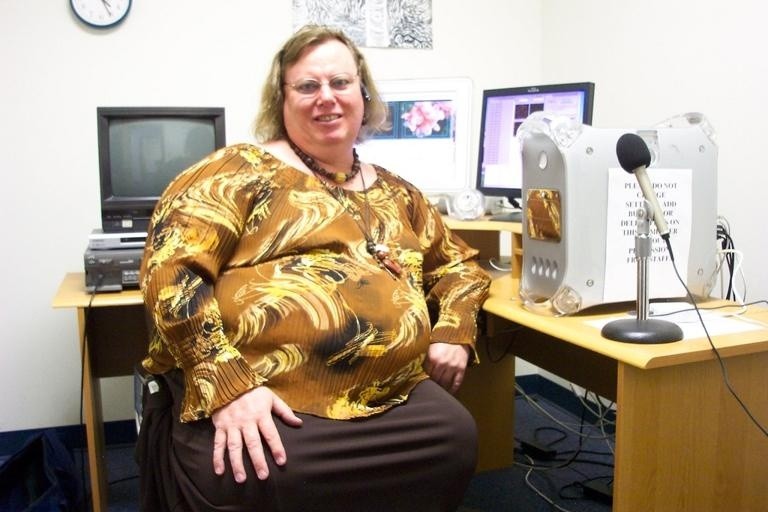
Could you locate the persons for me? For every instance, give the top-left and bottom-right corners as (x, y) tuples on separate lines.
(136, 20), (496, 510)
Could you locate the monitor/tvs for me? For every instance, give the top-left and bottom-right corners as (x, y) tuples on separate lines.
(97, 106), (224, 234)
(475, 81), (595, 223)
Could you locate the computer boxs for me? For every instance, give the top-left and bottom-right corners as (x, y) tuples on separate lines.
(518, 111), (721, 315)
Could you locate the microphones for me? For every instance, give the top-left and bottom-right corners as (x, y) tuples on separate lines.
(616, 133), (671, 241)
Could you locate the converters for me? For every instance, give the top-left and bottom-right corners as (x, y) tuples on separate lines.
(521, 440), (560, 462)
(582, 478), (612, 501)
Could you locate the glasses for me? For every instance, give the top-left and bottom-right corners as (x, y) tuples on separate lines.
(285, 72), (358, 99)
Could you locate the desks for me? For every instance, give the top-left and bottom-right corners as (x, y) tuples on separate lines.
(52, 218), (768, 512)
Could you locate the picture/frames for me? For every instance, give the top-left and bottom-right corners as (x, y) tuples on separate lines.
(398, 100), (454, 139)
(368, 101), (398, 139)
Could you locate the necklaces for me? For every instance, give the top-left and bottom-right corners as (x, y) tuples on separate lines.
(301, 160), (401, 281)
(288, 140), (362, 184)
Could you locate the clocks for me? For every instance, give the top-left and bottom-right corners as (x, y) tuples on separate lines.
(70, 0), (133, 29)
